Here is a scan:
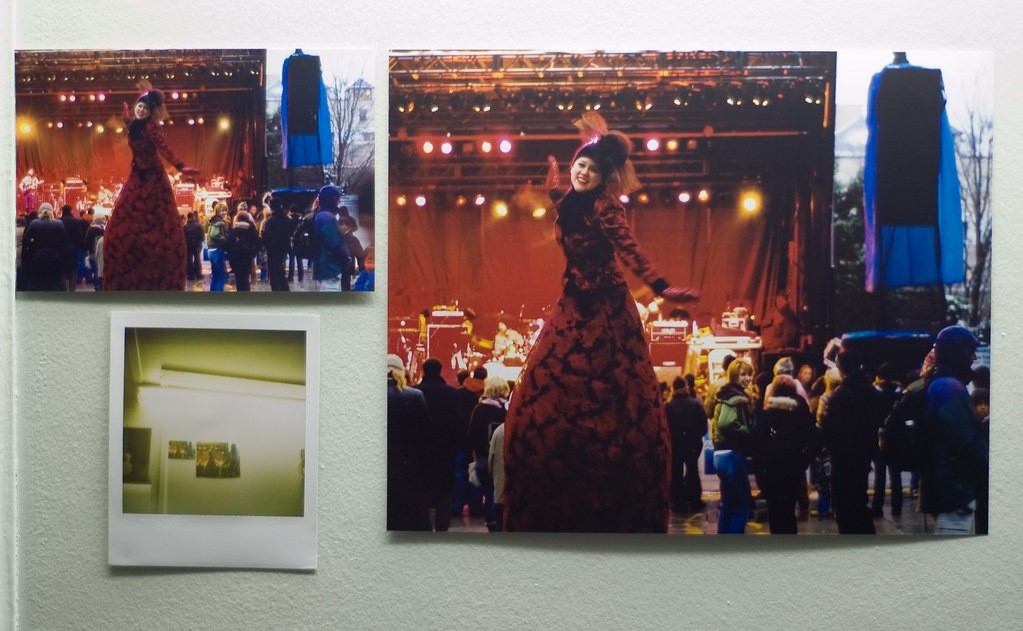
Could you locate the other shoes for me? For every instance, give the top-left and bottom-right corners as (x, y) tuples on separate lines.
(810, 509), (828, 516)
(870, 509), (882, 516)
(671, 506), (688, 513)
(257, 279), (266, 282)
(286, 278), (294, 281)
(187, 276), (195, 280)
(892, 508), (901, 514)
(797, 516), (808, 521)
(298, 278), (303, 281)
(692, 501), (705, 511)
(959, 508), (972, 515)
(197, 274), (204, 279)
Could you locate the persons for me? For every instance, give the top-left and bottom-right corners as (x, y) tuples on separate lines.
(179, 186), (375, 291)
(19, 168), (114, 291)
(501, 133), (672, 531)
(655, 326), (990, 535)
(103, 87), (187, 291)
(388, 315), (525, 531)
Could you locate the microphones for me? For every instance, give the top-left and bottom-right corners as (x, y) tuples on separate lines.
(455, 300), (459, 311)
(520, 304), (524, 317)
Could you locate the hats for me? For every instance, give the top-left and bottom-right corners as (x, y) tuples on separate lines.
(773, 357), (793, 375)
(388, 353), (404, 370)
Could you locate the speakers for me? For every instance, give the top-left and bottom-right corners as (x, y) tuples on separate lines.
(283, 54), (321, 137)
(63, 187), (87, 212)
(649, 342), (689, 374)
(429, 326), (470, 388)
(175, 195), (195, 212)
(865, 67), (944, 227)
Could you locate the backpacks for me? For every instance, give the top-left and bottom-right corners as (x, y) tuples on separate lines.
(877, 369), (951, 470)
(290, 208), (330, 258)
